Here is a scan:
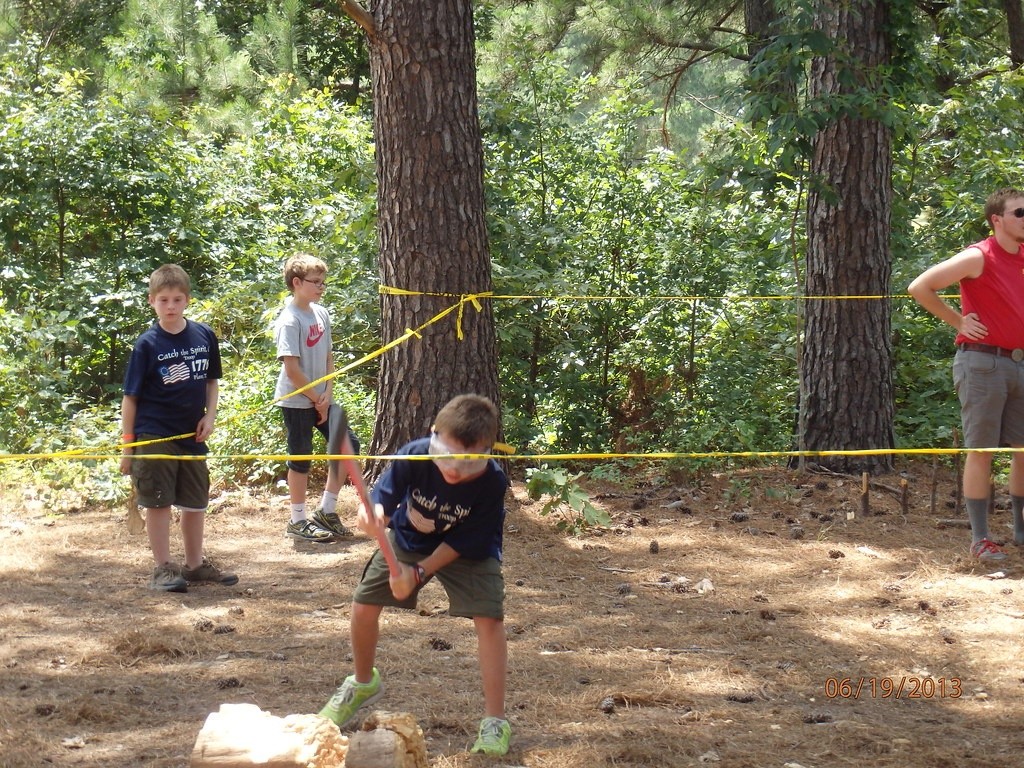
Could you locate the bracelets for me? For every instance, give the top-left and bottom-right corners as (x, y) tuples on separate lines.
(408, 561), (427, 589)
(122, 433), (135, 440)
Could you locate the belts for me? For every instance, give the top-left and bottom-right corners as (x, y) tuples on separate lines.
(958, 343), (1024, 362)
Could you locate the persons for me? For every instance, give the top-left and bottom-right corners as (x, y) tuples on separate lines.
(275, 256), (362, 541)
(907, 188), (1024, 560)
(318, 395), (513, 755)
(119, 263), (239, 592)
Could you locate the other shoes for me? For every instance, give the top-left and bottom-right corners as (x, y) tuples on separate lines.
(970, 539), (1008, 560)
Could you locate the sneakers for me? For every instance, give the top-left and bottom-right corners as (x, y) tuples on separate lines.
(317, 667), (385, 729)
(470, 717), (512, 756)
(152, 562), (187, 591)
(284, 519), (335, 541)
(311, 508), (354, 539)
(180, 557), (239, 587)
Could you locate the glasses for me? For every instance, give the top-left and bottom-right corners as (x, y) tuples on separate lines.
(428, 431), (491, 475)
(296, 275), (326, 287)
(996, 208), (1024, 217)
